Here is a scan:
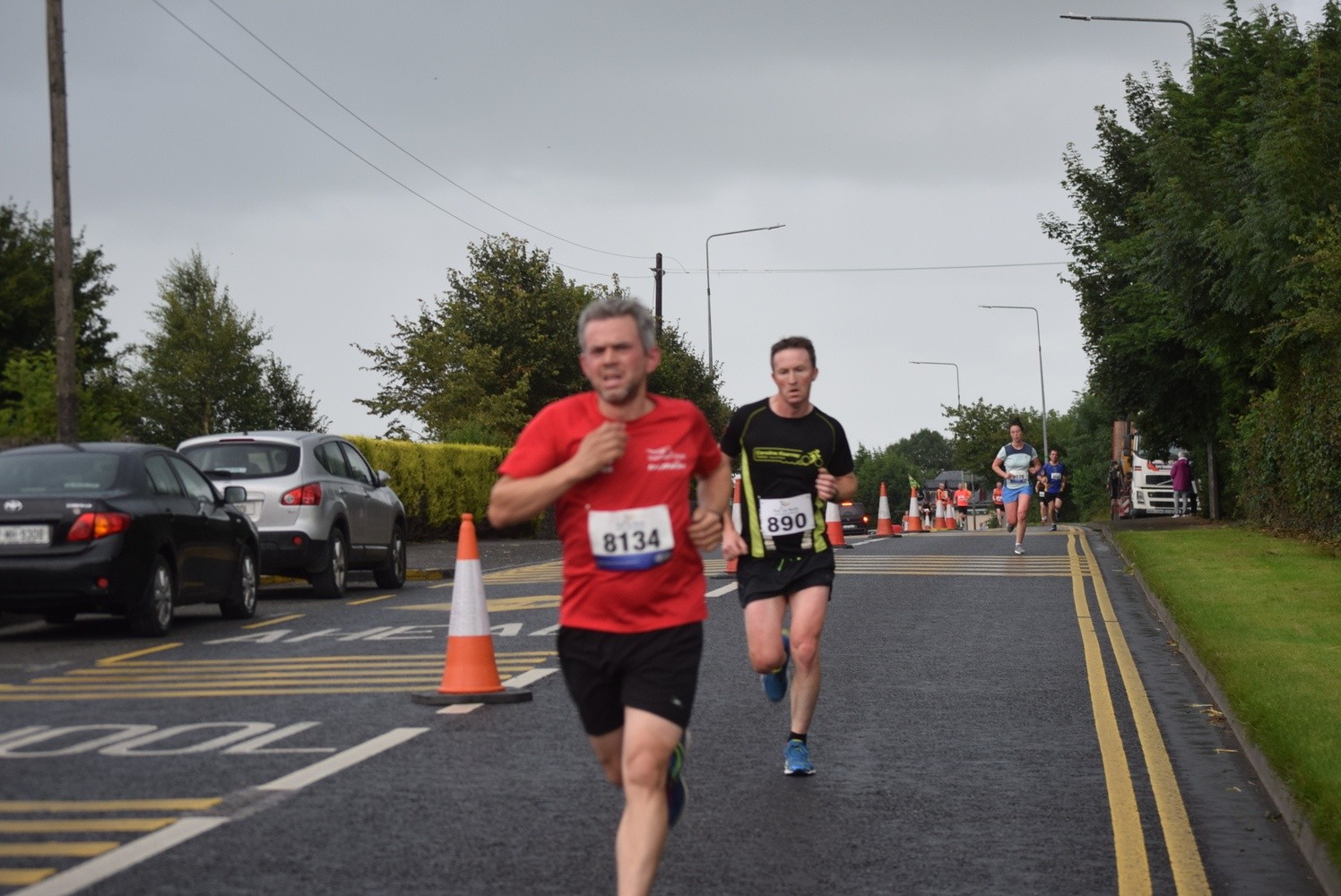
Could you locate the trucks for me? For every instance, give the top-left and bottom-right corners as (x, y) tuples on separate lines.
(1112, 411), (1202, 519)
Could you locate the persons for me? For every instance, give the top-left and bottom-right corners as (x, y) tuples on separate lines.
(1035, 450), (1067, 531)
(992, 480), (1005, 528)
(1170, 452), (1191, 518)
(720, 336), (858, 775)
(992, 417), (1041, 555)
(901, 510), (909, 530)
(488, 296), (732, 896)
(920, 482), (971, 530)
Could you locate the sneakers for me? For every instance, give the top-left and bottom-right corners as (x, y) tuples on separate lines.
(1014, 545), (1025, 554)
(760, 628), (791, 702)
(667, 730), (690, 829)
(1007, 523), (1014, 533)
(784, 739), (816, 775)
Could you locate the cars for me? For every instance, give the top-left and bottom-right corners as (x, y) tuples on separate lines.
(0, 442), (259, 637)
(839, 502), (868, 535)
(175, 432), (408, 598)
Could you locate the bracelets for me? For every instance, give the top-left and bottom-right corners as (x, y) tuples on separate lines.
(706, 509), (724, 523)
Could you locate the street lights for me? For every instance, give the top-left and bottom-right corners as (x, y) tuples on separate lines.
(979, 305), (1048, 465)
(707, 224), (785, 378)
(909, 361), (976, 530)
(1060, 12), (1197, 97)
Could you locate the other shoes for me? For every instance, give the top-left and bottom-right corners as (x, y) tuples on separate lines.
(1050, 524), (1056, 530)
(1172, 514), (1180, 518)
(1042, 517), (1047, 524)
(1182, 515), (1185, 517)
(1056, 512), (1060, 518)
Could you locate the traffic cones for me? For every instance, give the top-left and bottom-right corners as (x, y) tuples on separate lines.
(709, 475), (742, 579)
(410, 514), (533, 706)
(825, 501), (854, 549)
(932, 497), (958, 532)
(925, 511), (931, 530)
(900, 488), (930, 533)
(868, 482), (902, 538)
